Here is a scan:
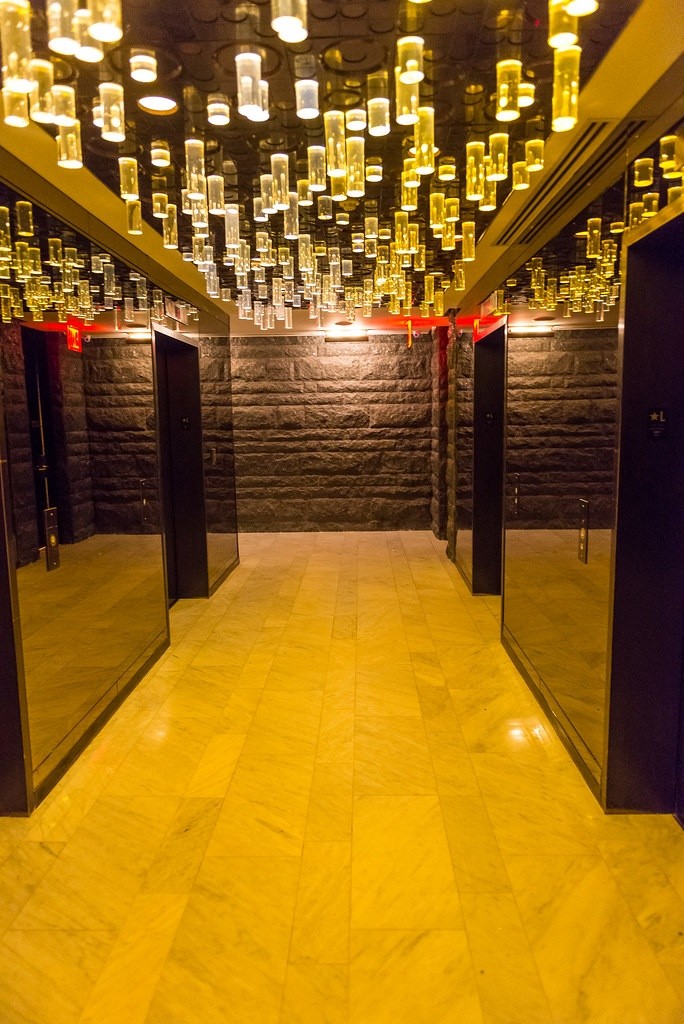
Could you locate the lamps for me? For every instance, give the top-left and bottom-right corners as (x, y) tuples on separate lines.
(0, 0), (599, 336)
(498, 115), (684, 322)
(2, 180), (206, 336)
